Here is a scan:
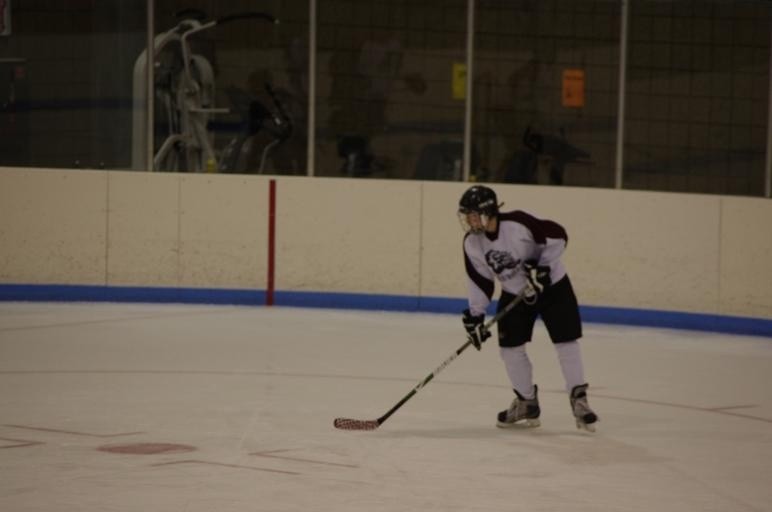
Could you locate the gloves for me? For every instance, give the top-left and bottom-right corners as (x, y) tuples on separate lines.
(522, 259), (551, 305)
(462, 310), (490, 350)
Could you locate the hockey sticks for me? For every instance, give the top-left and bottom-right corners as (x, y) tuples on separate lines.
(333, 283), (533, 431)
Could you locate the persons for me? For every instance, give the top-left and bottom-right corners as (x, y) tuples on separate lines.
(454, 186), (602, 425)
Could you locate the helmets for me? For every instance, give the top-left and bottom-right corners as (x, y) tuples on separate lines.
(457, 186), (498, 235)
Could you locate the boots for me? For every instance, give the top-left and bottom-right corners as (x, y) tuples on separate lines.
(569, 383), (598, 424)
(497, 384), (539, 422)
